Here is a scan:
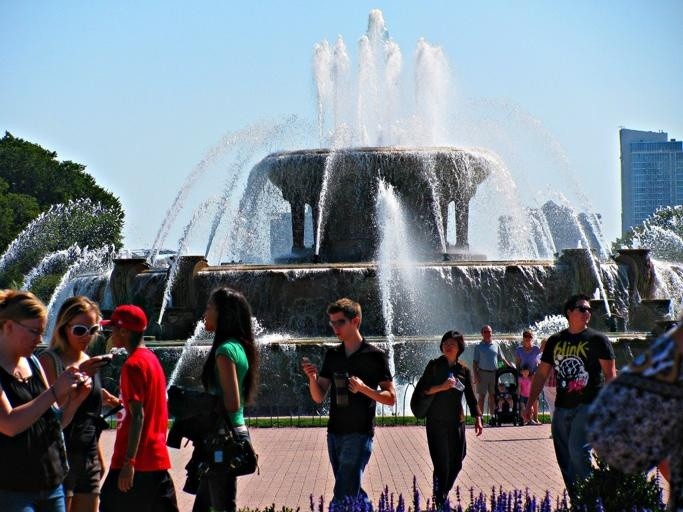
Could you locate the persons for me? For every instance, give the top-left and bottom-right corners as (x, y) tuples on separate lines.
(0, 288), (94, 512)
(473, 325), (558, 440)
(96, 304), (180, 512)
(425, 330), (482, 511)
(302, 299), (397, 512)
(36, 295), (106, 512)
(524, 294), (617, 507)
(588, 315), (683, 512)
(190, 288), (257, 512)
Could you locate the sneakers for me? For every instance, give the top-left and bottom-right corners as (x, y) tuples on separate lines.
(481, 415), (541, 428)
(498, 408), (513, 412)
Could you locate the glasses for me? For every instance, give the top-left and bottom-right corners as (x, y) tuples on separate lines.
(16, 322), (46, 335)
(329, 320), (348, 327)
(67, 322), (99, 337)
(574, 305), (592, 313)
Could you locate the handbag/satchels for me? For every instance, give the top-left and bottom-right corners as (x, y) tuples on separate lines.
(411, 377), (434, 419)
(585, 372), (683, 476)
(204, 438), (256, 477)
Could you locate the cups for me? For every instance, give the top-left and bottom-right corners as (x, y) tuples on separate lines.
(454, 378), (467, 391)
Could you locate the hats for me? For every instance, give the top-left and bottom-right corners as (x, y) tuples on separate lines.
(99, 305), (148, 332)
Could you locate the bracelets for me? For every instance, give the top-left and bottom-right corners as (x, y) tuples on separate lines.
(49, 384), (57, 400)
(123, 458), (136, 464)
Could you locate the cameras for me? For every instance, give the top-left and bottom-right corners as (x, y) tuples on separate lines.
(74, 372), (85, 391)
(91, 354), (113, 366)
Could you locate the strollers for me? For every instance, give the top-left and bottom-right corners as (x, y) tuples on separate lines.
(488, 366), (523, 427)
